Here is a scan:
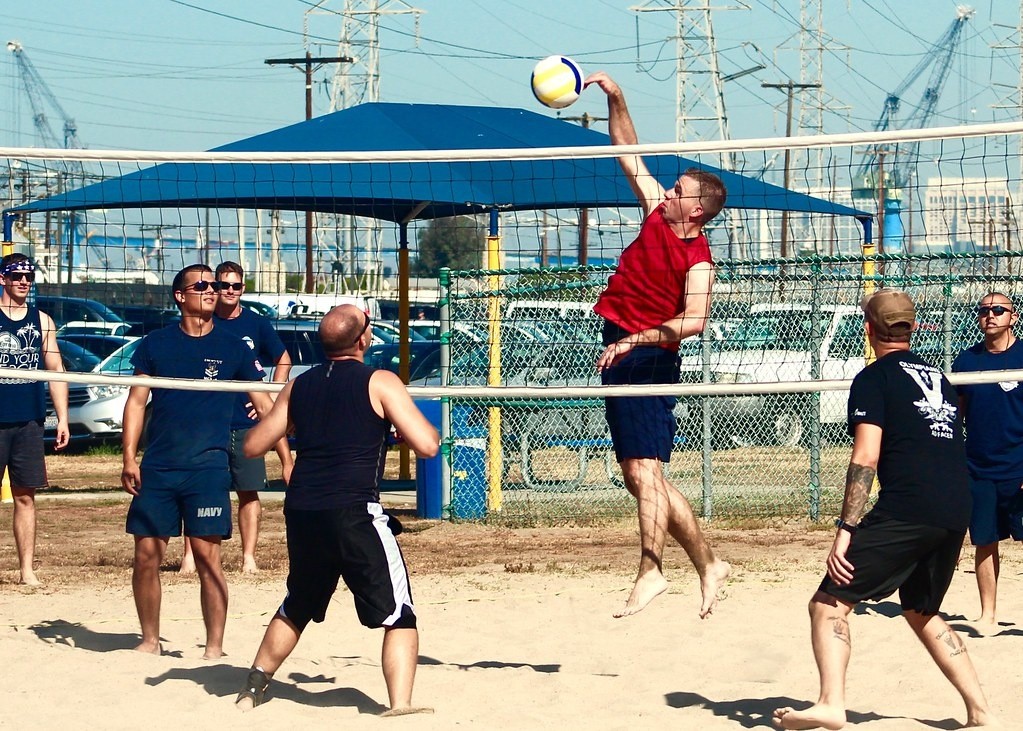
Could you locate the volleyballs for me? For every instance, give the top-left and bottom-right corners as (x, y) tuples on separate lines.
(530, 54), (585, 109)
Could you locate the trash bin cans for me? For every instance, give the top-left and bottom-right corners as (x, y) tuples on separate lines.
(415, 399), (486, 522)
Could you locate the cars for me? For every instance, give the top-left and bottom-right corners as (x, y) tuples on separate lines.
(33, 295), (1017, 447)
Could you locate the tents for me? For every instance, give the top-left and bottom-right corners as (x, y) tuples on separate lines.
(2, 102), (878, 507)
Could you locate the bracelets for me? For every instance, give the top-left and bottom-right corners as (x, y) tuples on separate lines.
(834, 519), (855, 533)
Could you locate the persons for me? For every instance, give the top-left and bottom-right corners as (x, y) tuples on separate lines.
(582, 69), (732, 618)
(0, 253), (70, 588)
(952, 291), (1023, 622)
(177, 259), (292, 573)
(121, 268), (294, 660)
(234, 304), (440, 718)
(771, 289), (991, 729)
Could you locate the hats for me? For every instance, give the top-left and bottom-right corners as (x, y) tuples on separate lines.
(863, 290), (916, 337)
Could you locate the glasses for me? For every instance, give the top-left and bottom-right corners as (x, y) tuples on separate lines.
(182, 281), (219, 292)
(220, 282), (243, 290)
(8, 272), (35, 281)
(353, 312), (370, 344)
(980, 306), (1011, 316)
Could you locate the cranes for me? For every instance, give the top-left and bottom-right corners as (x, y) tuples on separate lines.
(851, 0), (975, 285)
(6, 41), (86, 269)
